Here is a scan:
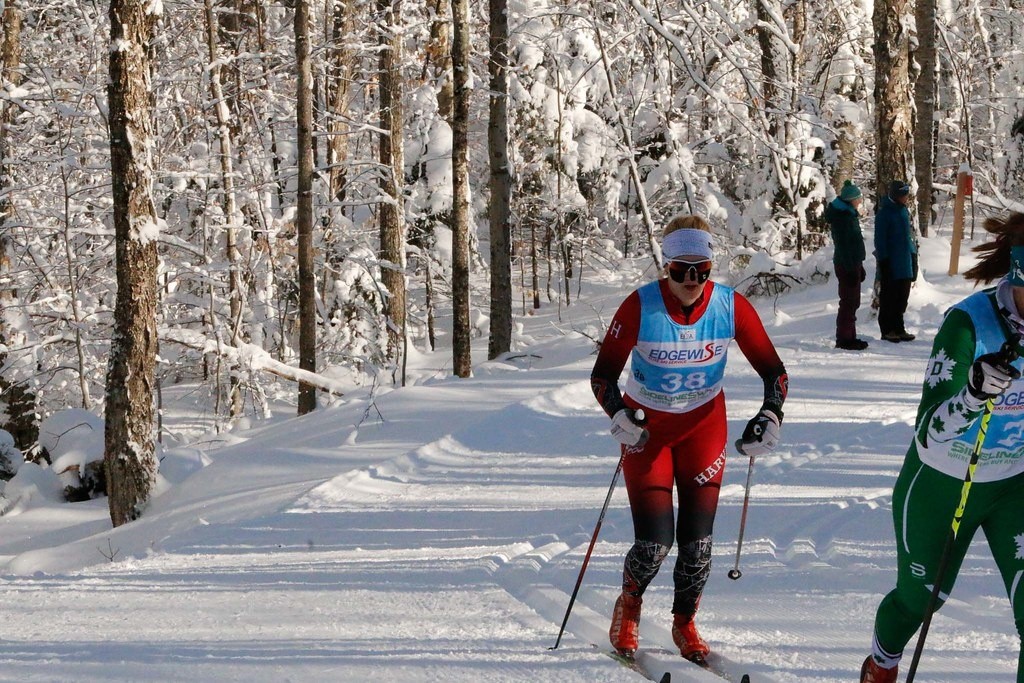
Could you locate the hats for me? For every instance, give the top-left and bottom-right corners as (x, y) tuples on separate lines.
(887, 180), (909, 200)
(840, 179), (861, 201)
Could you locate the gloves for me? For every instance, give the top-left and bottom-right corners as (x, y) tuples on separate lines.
(609, 407), (649, 453)
(967, 352), (1021, 401)
(853, 266), (866, 283)
(735, 410), (784, 456)
(877, 260), (896, 277)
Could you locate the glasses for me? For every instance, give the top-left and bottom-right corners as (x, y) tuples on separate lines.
(899, 185), (909, 190)
(665, 259), (713, 284)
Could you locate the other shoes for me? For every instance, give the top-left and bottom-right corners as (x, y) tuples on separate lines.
(834, 338), (868, 350)
(881, 330), (901, 343)
(899, 331), (915, 341)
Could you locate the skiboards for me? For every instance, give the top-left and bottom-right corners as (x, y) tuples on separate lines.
(590, 642), (750, 683)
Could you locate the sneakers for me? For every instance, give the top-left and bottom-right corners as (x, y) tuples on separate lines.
(860, 654), (899, 683)
(607, 591), (643, 661)
(671, 619), (710, 669)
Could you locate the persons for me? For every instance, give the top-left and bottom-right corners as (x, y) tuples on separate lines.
(824, 180), (868, 350)
(590, 216), (789, 661)
(873, 180), (919, 343)
(860, 214), (1024, 683)
